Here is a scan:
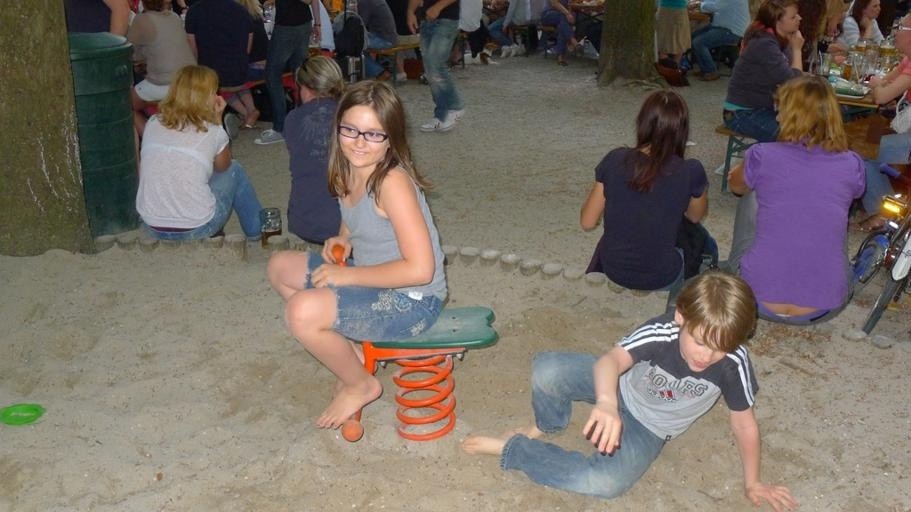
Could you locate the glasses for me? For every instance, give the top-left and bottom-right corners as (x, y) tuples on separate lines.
(337, 124), (388, 143)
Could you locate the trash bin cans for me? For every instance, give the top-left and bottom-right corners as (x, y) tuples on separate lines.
(68, 31), (141, 238)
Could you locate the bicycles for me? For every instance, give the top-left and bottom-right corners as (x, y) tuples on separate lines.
(860, 191), (911, 335)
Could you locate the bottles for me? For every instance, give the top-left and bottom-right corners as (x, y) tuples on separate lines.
(331, 243), (347, 270)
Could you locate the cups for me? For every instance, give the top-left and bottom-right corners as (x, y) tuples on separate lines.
(264, 6), (273, 23)
(817, 37), (904, 103)
(259, 207), (283, 249)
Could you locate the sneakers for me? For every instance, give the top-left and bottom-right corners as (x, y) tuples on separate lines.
(377, 71), (391, 81)
(500, 46), (520, 57)
(395, 72), (407, 82)
(481, 50), (496, 64)
(575, 44), (584, 58)
(694, 70), (720, 81)
(420, 117), (441, 132)
(254, 129), (285, 145)
(438, 110), (464, 131)
(558, 55), (568, 66)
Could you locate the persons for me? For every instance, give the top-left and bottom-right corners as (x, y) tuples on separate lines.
(283, 56), (354, 256)
(266, 82), (443, 430)
(135, 65), (264, 241)
(459, 271), (798, 512)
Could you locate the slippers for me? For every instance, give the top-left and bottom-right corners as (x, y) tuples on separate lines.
(239, 123), (264, 129)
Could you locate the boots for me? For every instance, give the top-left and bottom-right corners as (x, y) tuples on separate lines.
(729, 159), (745, 197)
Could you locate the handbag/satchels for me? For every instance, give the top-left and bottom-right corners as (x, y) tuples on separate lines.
(890, 103), (911, 134)
(655, 59), (689, 86)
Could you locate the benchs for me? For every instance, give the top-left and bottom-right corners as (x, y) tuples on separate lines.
(711, 23), (909, 224)
(450, 1), (718, 70)
(128, 0), (466, 127)
